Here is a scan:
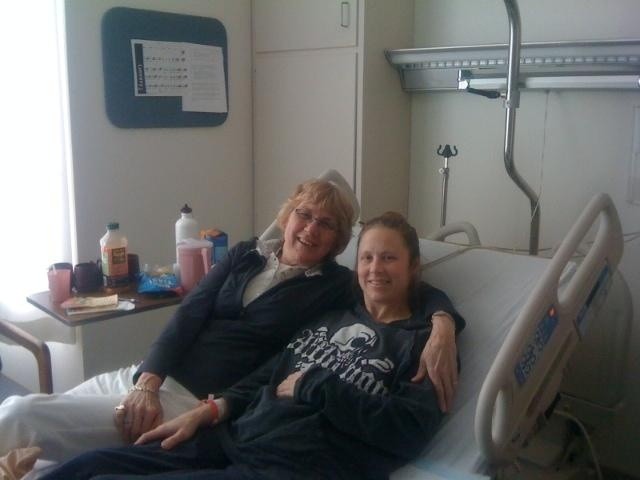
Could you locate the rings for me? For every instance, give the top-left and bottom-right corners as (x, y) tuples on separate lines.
(114, 405), (127, 412)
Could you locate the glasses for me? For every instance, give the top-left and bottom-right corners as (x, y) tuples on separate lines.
(293, 209), (336, 230)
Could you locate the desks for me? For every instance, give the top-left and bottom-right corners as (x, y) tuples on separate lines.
(27, 280), (184, 327)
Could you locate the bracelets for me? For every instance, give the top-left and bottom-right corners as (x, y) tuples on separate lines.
(129, 384), (160, 394)
(202, 399), (219, 428)
(431, 312), (457, 324)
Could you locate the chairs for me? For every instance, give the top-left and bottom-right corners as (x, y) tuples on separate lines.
(0, 319), (53, 404)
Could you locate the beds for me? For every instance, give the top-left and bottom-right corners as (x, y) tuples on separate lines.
(34, 168), (625, 480)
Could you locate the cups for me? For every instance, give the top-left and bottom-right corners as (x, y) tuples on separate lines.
(75, 264), (98, 290)
(48, 270), (71, 303)
(128, 253), (141, 281)
(48, 262), (74, 289)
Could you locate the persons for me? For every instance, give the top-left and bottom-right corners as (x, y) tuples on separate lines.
(32, 208), (449, 480)
(0, 181), (467, 475)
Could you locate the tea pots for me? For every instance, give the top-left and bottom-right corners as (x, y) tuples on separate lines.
(176, 239), (213, 290)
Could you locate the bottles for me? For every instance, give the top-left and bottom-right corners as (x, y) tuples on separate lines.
(174, 203), (202, 242)
(206, 232), (228, 260)
(100, 221), (130, 292)
